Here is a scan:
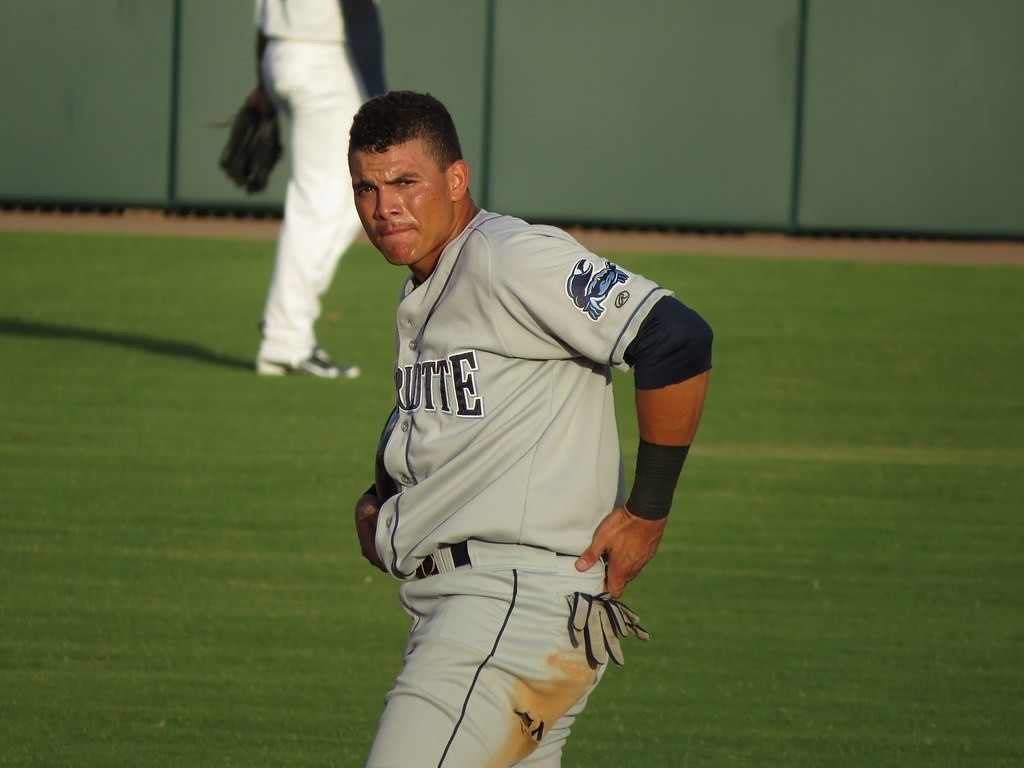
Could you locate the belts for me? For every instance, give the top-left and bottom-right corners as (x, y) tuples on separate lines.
(415, 541), (471, 578)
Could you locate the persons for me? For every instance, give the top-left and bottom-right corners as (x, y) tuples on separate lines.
(219, 0), (387, 379)
(347, 89), (715, 767)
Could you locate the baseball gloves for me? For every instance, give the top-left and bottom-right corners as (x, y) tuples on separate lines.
(216, 91), (284, 196)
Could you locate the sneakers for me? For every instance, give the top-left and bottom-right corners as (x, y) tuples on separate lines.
(254, 349), (361, 378)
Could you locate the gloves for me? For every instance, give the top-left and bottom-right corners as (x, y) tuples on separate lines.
(571, 591), (652, 667)
